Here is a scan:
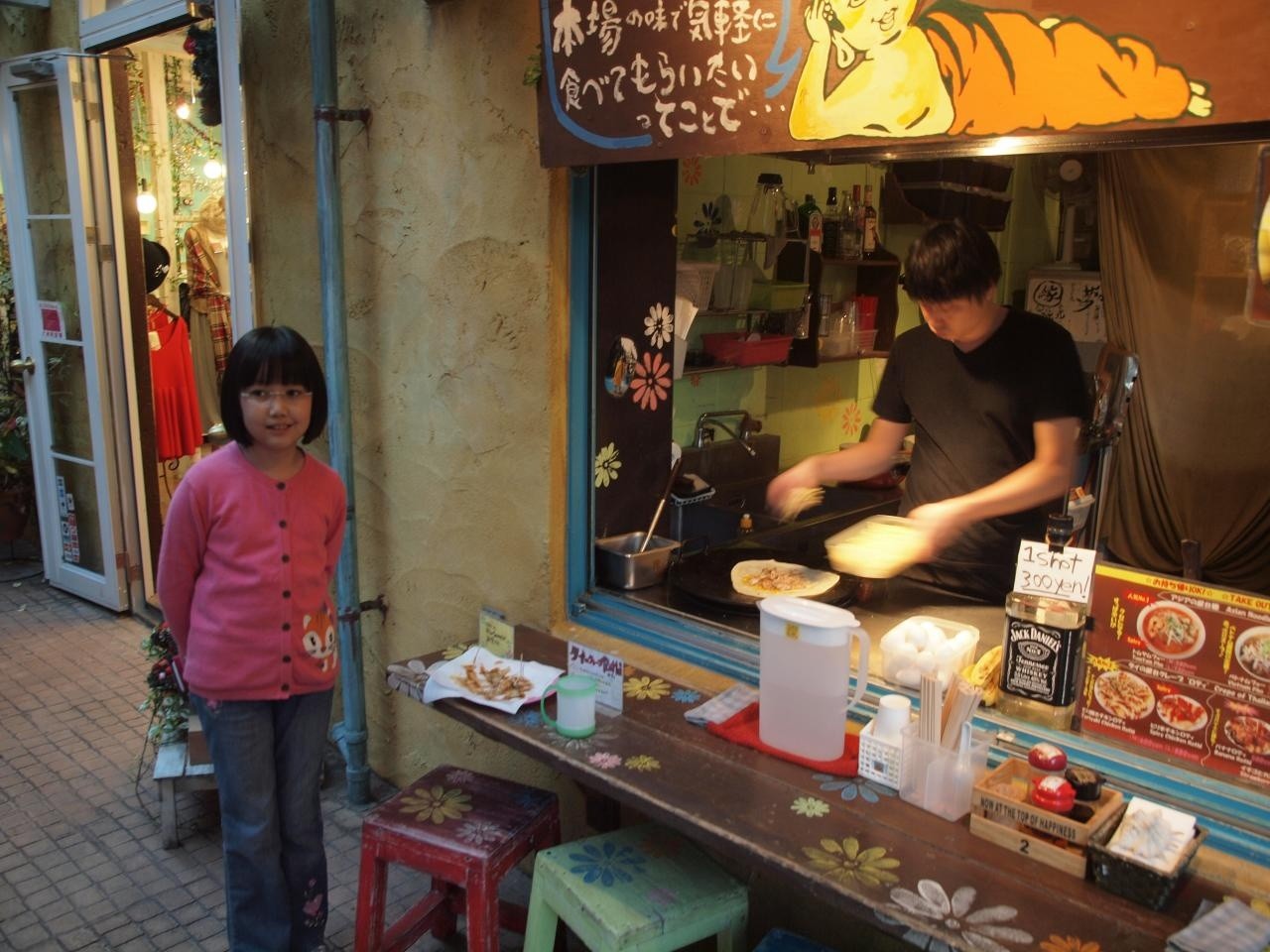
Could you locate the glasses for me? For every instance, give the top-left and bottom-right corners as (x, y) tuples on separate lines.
(238, 387), (312, 406)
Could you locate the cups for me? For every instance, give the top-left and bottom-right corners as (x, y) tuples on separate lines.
(541, 674), (597, 738)
(819, 290), (833, 339)
(873, 693), (911, 746)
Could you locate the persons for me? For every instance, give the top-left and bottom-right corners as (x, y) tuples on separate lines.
(764, 217), (1092, 617)
(156, 325), (346, 952)
(186, 194), (232, 435)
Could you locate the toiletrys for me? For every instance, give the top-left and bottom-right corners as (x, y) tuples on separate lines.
(734, 510), (755, 536)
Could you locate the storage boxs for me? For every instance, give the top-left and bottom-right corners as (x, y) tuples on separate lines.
(881, 615), (980, 692)
(899, 718), (993, 821)
(1087, 802), (1209, 909)
(749, 281), (809, 311)
(188, 715), (213, 765)
(970, 756), (1123, 878)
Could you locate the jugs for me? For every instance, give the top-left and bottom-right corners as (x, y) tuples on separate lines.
(757, 594), (870, 761)
(745, 173), (799, 281)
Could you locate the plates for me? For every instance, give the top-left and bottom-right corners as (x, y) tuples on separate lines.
(1137, 599), (1206, 659)
(1224, 716), (1270, 756)
(1094, 670), (1155, 719)
(1235, 625), (1270, 682)
(522, 676), (559, 705)
(1157, 693), (1208, 731)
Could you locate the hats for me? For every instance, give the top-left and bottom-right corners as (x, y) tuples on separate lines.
(142, 237), (169, 294)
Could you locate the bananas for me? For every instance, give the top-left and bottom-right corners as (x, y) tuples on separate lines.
(961, 646), (1001, 707)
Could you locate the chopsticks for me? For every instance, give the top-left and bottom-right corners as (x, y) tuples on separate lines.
(917, 673), (984, 750)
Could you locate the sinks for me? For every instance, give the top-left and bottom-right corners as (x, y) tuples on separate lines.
(660, 502), (788, 556)
(717, 478), (874, 522)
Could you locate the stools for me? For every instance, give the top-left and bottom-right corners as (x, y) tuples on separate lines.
(524, 821), (750, 952)
(356, 763), (566, 952)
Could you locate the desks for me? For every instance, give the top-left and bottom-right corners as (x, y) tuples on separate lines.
(153, 733), (217, 850)
(386, 624), (1270, 952)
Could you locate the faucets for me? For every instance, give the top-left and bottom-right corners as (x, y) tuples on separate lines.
(694, 411), (764, 462)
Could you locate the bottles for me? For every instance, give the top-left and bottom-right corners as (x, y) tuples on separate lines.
(998, 513), (1087, 718)
(798, 183), (878, 261)
(1026, 741), (1106, 850)
(735, 513), (755, 538)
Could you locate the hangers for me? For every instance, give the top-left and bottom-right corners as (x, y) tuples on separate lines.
(147, 293), (178, 321)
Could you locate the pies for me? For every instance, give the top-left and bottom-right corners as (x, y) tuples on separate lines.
(731, 558), (842, 597)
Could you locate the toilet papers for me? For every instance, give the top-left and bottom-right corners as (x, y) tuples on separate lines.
(670, 442), (682, 471)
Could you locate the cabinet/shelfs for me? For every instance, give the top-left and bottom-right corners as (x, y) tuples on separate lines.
(682, 234), (899, 375)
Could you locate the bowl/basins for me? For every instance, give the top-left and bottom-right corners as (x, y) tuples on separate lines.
(595, 529), (681, 589)
(822, 513), (933, 579)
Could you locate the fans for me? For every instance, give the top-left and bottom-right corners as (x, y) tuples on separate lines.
(1031, 152), (1099, 271)
